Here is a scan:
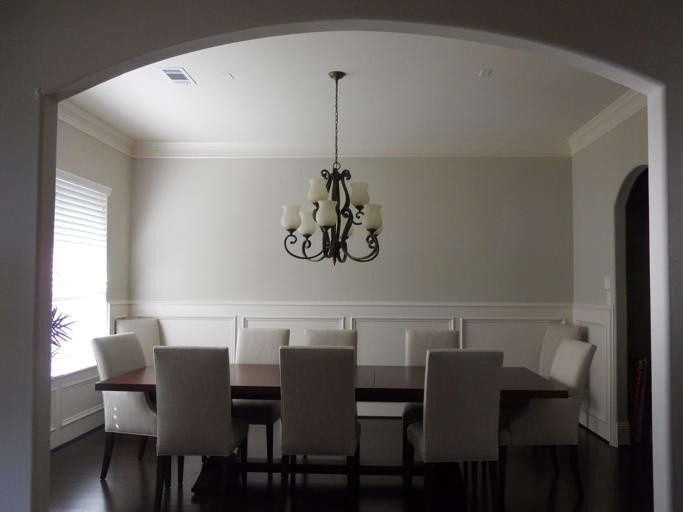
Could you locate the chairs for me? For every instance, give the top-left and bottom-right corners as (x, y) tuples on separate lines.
(404, 348), (506, 510)
(303, 325), (360, 368)
(231, 325), (291, 485)
(114, 319), (164, 368)
(152, 343), (250, 510)
(499, 322), (597, 511)
(278, 344), (364, 511)
(400, 325), (463, 493)
(90, 332), (186, 487)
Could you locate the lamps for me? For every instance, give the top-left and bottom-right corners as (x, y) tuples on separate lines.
(281, 71), (383, 266)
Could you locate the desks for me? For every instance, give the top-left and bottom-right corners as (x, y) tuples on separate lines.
(94, 362), (571, 511)
